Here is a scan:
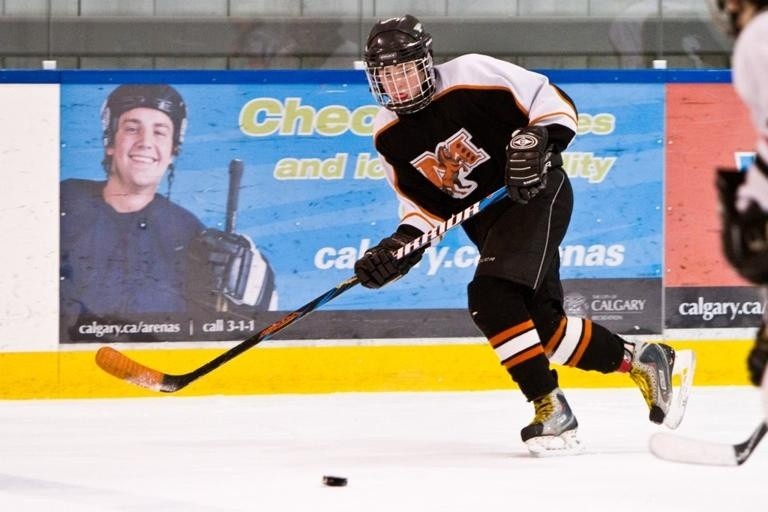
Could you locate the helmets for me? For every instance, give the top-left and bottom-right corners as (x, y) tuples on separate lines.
(100, 84), (187, 146)
(363, 14), (433, 68)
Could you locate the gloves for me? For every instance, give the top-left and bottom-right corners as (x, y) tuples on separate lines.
(173, 228), (276, 319)
(504, 125), (549, 206)
(715, 153), (768, 286)
(354, 223), (431, 289)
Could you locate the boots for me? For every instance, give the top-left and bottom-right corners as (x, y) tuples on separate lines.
(520, 385), (579, 442)
(621, 340), (676, 426)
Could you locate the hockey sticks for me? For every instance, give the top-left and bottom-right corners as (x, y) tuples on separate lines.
(649, 421), (768, 464)
(95, 150), (563, 394)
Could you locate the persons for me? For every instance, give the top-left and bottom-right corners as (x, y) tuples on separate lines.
(707, 0), (768, 389)
(354, 15), (674, 442)
(61, 83), (279, 313)
